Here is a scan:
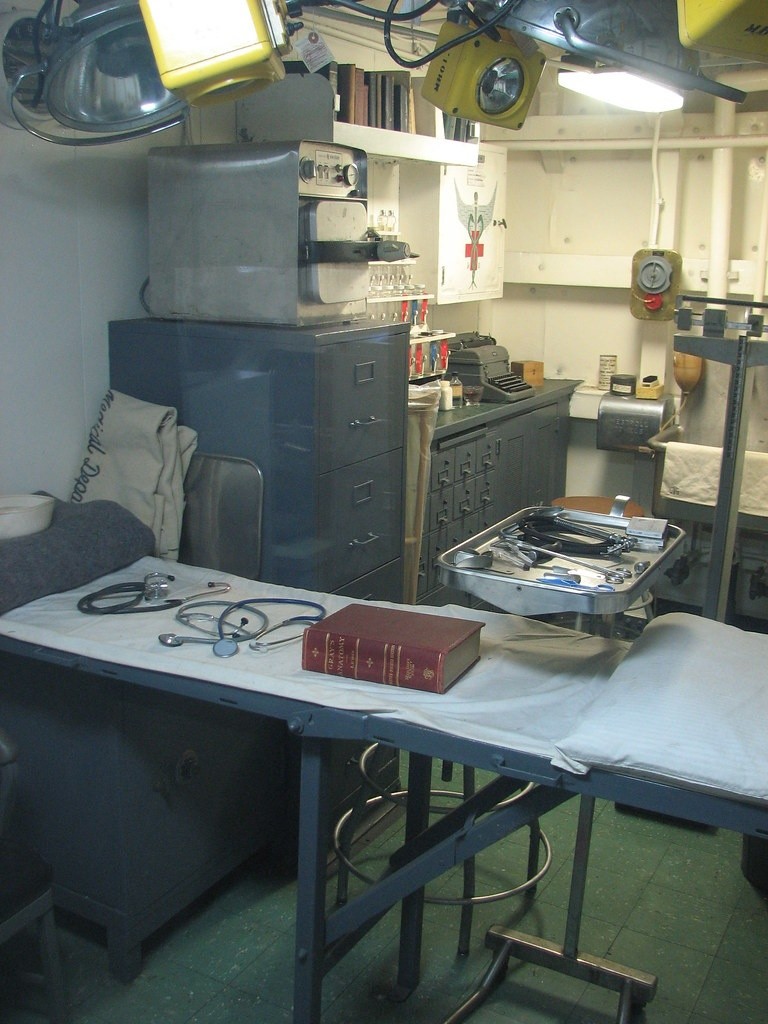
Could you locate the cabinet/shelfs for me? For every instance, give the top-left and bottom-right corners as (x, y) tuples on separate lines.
(110, 318), (410, 607)
(236, 59), (504, 305)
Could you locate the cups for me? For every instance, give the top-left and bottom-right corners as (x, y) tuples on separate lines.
(463, 385), (484, 407)
(610, 374), (637, 396)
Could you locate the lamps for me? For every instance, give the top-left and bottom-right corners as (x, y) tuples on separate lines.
(557, 50), (684, 113)
(421, 9), (546, 131)
(676, 0), (768, 64)
(138, 0), (305, 109)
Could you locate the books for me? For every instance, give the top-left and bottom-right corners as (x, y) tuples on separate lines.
(301, 603), (485, 694)
(282, 60), (475, 142)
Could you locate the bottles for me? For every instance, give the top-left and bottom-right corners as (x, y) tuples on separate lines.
(439, 381), (453, 410)
(377, 210), (396, 232)
(449, 372), (463, 408)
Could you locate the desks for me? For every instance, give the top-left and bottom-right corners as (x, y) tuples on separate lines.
(409, 378), (582, 615)
(0, 556), (768, 1024)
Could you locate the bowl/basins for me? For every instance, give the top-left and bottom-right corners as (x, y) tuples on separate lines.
(551, 495), (644, 517)
(0, 494), (56, 540)
